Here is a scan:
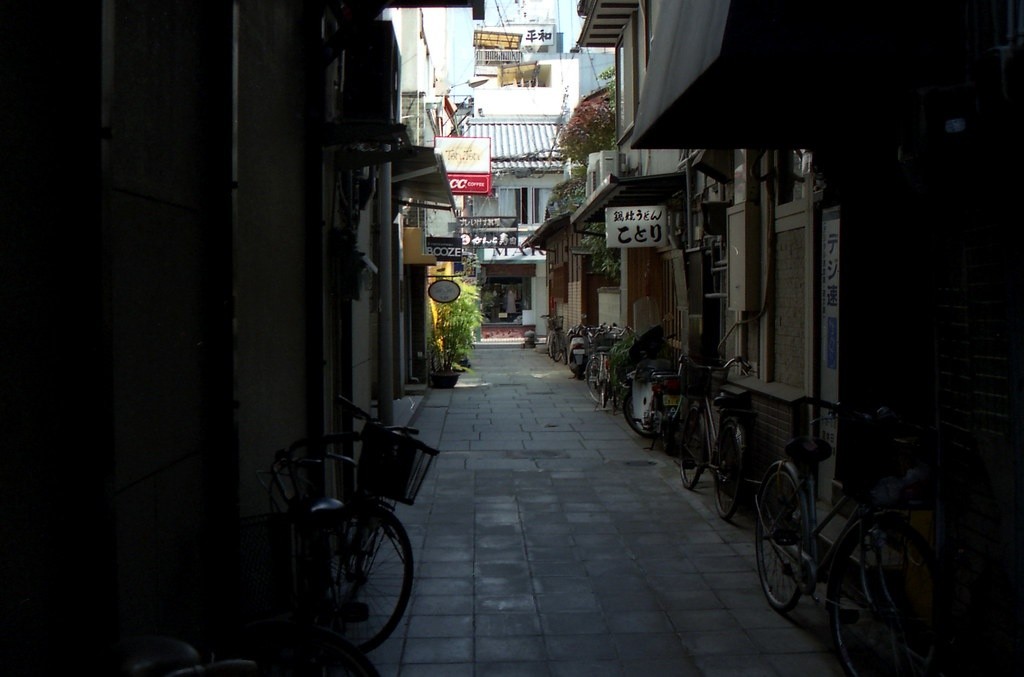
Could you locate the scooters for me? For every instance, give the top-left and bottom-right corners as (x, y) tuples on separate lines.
(564, 314), (593, 380)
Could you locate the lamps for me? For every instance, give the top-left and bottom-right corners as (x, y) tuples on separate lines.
(446, 75), (490, 97)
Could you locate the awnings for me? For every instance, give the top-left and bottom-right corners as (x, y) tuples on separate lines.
(395, 143), (459, 212)
(630, 0), (991, 152)
(568, 170), (691, 227)
(403, 227), (437, 266)
(519, 211), (574, 251)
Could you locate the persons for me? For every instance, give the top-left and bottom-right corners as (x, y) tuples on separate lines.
(504, 289), (518, 314)
(491, 290), (501, 323)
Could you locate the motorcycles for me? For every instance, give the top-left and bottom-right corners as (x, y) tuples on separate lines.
(618, 322), (683, 456)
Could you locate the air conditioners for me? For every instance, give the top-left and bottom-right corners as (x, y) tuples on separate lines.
(585, 151), (618, 198)
(341, 20), (400, 123)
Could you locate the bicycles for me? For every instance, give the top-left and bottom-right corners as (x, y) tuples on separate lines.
(755, 394), (956, 677)
(677, 353), (751, 522)
(539, 313), (565, 362)
(585, 326), (616, 412)
(114, 404), (442, 677)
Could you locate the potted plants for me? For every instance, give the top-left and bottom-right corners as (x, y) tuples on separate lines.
(429, 278), (485, 390)
